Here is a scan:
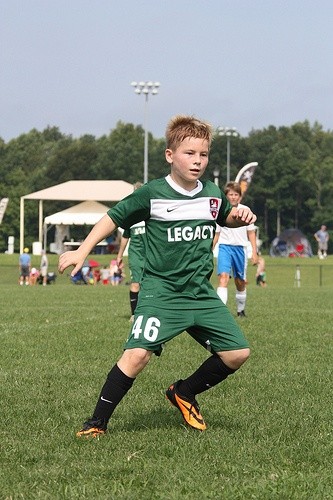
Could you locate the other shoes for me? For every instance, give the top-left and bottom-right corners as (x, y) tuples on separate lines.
(128, 315), (135, 322)
(237, 310), (248, 319)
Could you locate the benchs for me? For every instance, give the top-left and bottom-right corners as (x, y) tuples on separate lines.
(64, 241), (109, 256)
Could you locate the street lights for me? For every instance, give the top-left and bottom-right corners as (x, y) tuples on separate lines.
(218, 126), (239, 185)
(130, 81), (161, 187)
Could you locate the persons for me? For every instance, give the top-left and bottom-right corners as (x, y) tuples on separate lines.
(118, 182), (145, 321)
(256, 252), (266, 287)
(30, 267), (40, 286)
(213, 182), (257, 318)
(40, 251), (47, 286)
(314, 225), (329, 260)
(58, 114), (256, 436)
(19, 248), (31, 285)
(70, 259), (127, 286)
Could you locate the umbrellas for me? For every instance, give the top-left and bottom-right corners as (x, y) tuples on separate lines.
(81, 259), (99, 268)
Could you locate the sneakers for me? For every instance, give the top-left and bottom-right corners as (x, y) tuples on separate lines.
(165, 379), (207, 431)
(76, 418), (109, 438)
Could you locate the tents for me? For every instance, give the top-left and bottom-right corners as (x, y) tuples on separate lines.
(270, 229), (313, 258)
(44, 201), (125, 255)
(20, 180), (134, 255)
